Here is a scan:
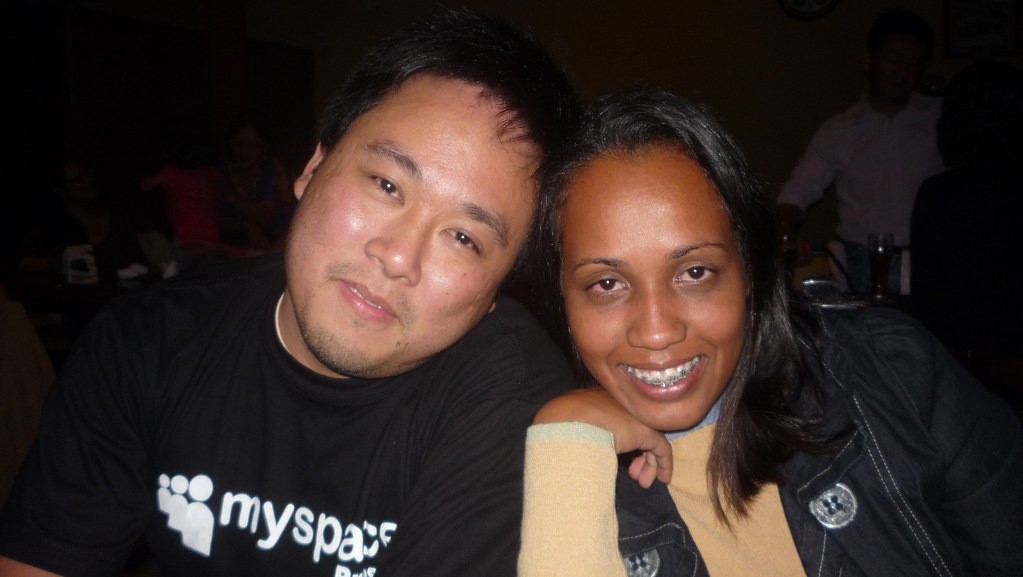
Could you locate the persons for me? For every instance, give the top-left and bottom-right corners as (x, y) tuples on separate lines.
(516, 90), (1022, 576)
(14, 110), (304, 283)
(770, 10), (939, 304)
(0, 0), (593, 577)
(909, 11), (1023, 389)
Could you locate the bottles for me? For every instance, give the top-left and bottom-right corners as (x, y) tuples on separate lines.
(775, 219), (818, 297)
(869, 232), (902, 305)
